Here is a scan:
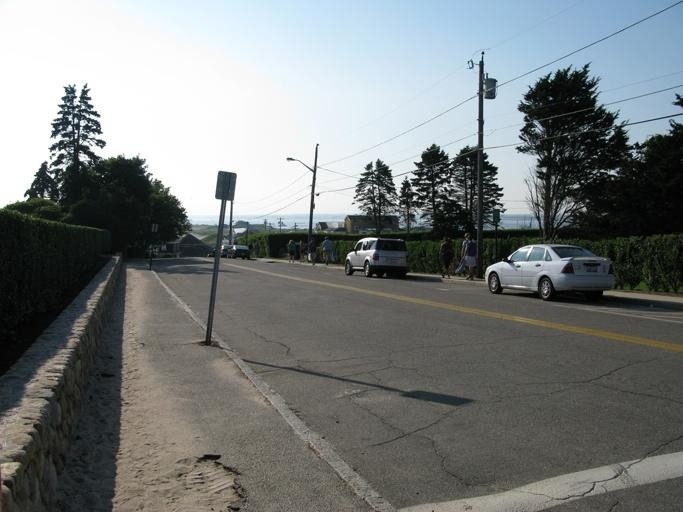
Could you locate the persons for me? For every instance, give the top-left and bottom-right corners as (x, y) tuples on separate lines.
(439, 236), (451, 280)
(287, 235), (332, 267)
(463, 235), (476, 281)
(454, 232), (475, 280)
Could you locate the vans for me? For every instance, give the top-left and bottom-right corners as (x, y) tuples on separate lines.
(345, 237), (408, 277)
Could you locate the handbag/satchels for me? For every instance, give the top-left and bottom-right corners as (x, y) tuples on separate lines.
(454, 263), (466, 275)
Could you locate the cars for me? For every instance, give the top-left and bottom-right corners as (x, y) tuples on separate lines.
(485, 243), (618, 300)
(221, 244), (250, 260)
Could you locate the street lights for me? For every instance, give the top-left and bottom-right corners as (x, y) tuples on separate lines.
(287, 144), (319, 262)
(467, 50), (485, 278)
